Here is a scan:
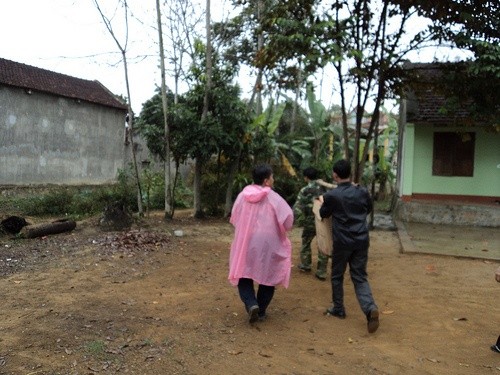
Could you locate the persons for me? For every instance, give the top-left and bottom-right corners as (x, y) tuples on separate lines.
(227, 164), (294, 326)
(319, 159), (380, 334)
(317, 172), (359, 189)
(291, 166), (329, 279)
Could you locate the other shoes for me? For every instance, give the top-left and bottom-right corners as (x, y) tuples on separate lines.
(327, 305), (346, 317)
(257, 312), (269, 322)
(297, 264), (312, 273)
(248, 305), (259, 324)
(368, 307), (380, 333)
(315, 269), (327, 280)
